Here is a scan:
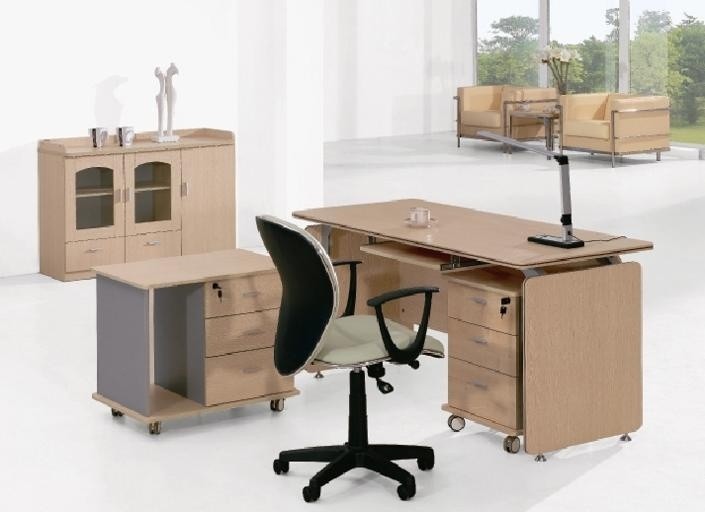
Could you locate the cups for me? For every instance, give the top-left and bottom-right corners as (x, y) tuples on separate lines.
(116, 126), (135, 147)
(88, 128), (108, 149)
(409, 207), (431, 224)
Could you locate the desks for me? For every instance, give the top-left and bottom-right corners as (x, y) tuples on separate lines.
(292, 198), (653, 462)
(89, 247), (301, 435)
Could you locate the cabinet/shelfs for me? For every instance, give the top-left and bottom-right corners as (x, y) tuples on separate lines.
(38, 128), (237, 282)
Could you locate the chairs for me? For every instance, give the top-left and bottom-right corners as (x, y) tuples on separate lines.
(556, 92), (671, 168)
(255, 214), (444, 502)
(453, 84), (556, 153)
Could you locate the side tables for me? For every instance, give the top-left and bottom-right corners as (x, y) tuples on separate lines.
(508, 110), (559, 160)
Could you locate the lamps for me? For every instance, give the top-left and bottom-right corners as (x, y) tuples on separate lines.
(476, 130), (585, 249)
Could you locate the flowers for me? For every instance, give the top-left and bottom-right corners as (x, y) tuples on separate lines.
(534, 45), (579, 95)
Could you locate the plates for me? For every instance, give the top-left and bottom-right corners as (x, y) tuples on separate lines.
(403, 216), (439, 227)
